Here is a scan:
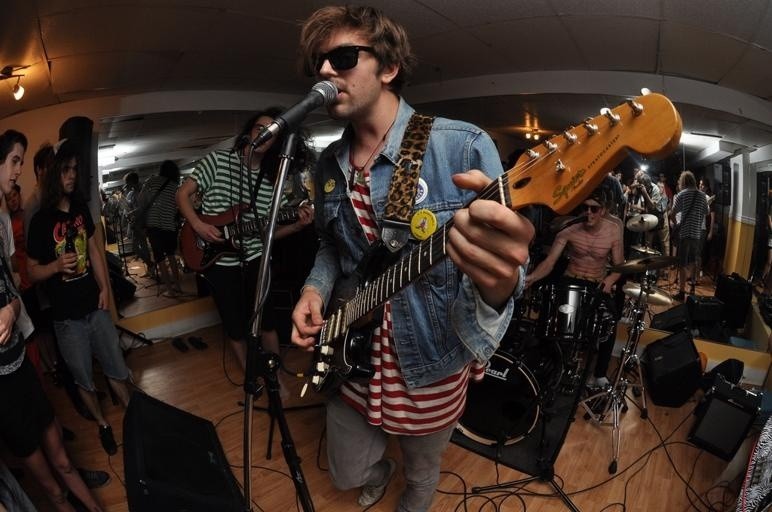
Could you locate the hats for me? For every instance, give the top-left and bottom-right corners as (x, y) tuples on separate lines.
(44, 136), (70, 166)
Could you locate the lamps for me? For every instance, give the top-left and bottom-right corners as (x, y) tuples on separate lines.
(1, 75), (25, 101)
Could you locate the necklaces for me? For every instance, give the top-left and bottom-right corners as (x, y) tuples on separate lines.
(348, 119), (395, 190)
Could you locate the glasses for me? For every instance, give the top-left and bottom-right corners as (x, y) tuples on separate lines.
(303, 46), (375, 76)
(582, 204), (602, 213)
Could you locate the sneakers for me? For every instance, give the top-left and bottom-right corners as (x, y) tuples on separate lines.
(671, 291), (684, 302)
(358, 457), (397, 507)
(99, 424), (117, 456)
(79, 468), (111, 488)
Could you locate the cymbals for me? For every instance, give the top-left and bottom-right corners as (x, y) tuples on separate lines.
(631, 244), (660, 257)
(615, 255), (674, 269)
(627, 214), (658, 231)
(613, 281), (672, 305)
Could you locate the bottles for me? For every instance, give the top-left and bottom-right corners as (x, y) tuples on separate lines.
(65, 236), (78, 271)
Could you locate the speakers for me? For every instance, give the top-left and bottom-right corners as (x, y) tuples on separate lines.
(452, 319), (581, 478)
(107, 265), (136, 311)
(123, 389), (243, 512)
(688, 384), (758, 461)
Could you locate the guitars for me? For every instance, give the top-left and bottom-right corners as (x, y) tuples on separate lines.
(308, 88), (682, 398)
(181, 202), (315, 273)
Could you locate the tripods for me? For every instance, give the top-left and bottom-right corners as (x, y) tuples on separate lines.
(578, 320), (647, 475)
(470, 331), (581, 512)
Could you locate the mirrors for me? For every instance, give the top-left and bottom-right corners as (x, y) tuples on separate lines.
(97, 112), (345, 321)
(410, 94), (772, 355)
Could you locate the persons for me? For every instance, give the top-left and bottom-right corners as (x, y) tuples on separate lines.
(174, 104), (315, 377)
(287, 0), (537, 512)
(0, 127), (135, 511)
(122, 159), (182, 298)
(603, 168), (715, 302)
(524, 188), (624, 388)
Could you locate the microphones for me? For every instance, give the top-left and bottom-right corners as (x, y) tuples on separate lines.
(252, 81), (337, 147)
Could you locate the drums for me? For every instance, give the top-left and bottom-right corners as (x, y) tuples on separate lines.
(537, 277), (604, 340)
(456, 321), (565, 448)
